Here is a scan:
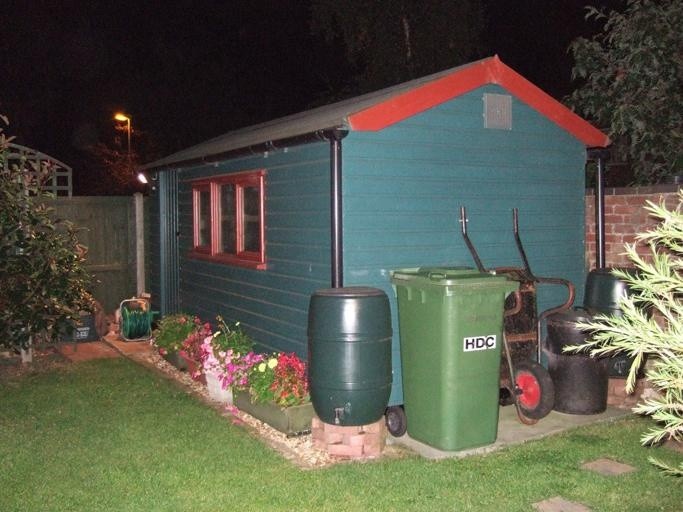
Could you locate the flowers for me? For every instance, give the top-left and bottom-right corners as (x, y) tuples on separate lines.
(146, 310), (215, 363)
(189, 314), (260, 381)
(217, 347), (310, 412)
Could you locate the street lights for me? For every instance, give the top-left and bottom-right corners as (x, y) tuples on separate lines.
(114, 113), (131, 163)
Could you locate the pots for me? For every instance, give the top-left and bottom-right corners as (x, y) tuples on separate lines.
(547, 305), (605, 354)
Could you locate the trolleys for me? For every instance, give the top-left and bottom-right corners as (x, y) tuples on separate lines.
(451, 206), (575, 424)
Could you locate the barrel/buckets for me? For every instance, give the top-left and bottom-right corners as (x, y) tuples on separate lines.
(307, 289), (394, 428)
(583, 269), (650, 379)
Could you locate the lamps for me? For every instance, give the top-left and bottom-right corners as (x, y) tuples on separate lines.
(135, 170), (157, 184)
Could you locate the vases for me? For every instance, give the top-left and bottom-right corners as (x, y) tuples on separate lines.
(157, 343), (187, 371)
(202, 372), (231, 401)
(183, 356), (206, 385)
(232, 386), (313, 439)
